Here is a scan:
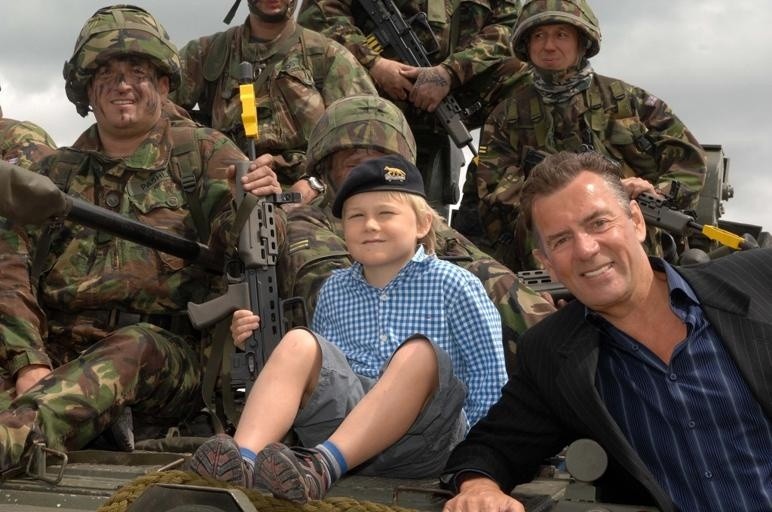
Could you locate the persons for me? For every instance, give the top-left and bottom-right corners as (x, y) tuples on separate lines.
(0, 0), (288, 482)
(439, 153), (772, 512)
(173, 1), (707, 479)
(189, 154), (508, 505)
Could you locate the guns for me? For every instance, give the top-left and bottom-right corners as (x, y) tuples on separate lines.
(187, 60), (311, 395)
(518, 145), (759, 251)
(358, 0), (482, 156)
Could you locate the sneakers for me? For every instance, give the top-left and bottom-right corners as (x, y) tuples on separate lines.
(84, 408), (136, 453)
(187, 433), (252, 492)
(255, 440), (330, 504)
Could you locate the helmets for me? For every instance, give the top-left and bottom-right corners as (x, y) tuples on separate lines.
(63, 3), (183, 117)
(306, 94), (417, 171)
(509, 0), (601, 62)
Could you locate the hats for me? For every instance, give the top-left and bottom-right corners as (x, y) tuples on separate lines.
(333, 154), (427, 218)
